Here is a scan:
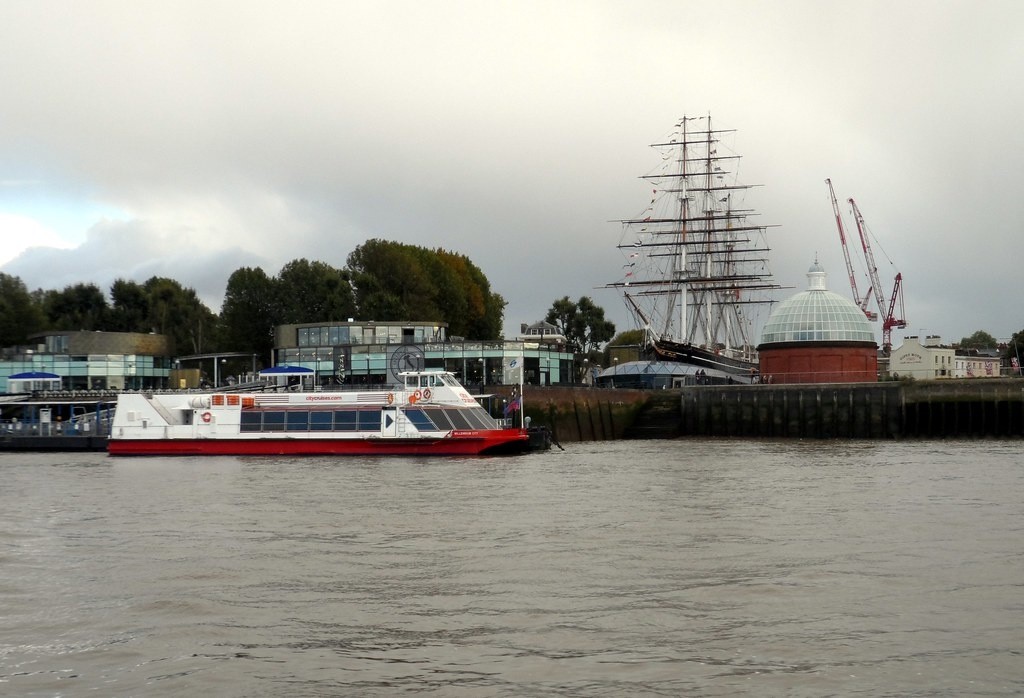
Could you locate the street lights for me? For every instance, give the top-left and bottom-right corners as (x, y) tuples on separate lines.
(545, 358), (550, 385)
(478, 358), (483, 387)
(317, 357), (321, 386)
(614, 357), (618, 387)
(366, 355), (370, 389)
(582, 358), (588, 387)
(415, 354), (420, 371)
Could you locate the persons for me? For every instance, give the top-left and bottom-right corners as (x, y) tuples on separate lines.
(762, 374), (775, 384)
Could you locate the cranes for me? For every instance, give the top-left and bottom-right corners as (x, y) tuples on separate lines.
(824, 178), (907, 363)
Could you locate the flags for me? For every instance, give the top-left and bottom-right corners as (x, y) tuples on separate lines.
(734, 290), (740, 300)
(985, 361), (993, 375)
(965, 362), (974, 377)
(505, 354), (520, 370)
(1011, 357), (1020, 372)
(622, 119), (684, 277)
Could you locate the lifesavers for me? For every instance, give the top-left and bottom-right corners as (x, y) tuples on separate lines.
(423, 389), (431, 399)
(388, 394), (393, 404)
(414, 390), (422, 400)
(203, 412), (211, 422)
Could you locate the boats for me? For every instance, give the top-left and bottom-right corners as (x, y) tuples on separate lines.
(474, 394), (556, 451)
(105, 371), (530, 455)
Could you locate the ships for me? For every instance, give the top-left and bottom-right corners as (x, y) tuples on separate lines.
(592, 108), (798, 375)
